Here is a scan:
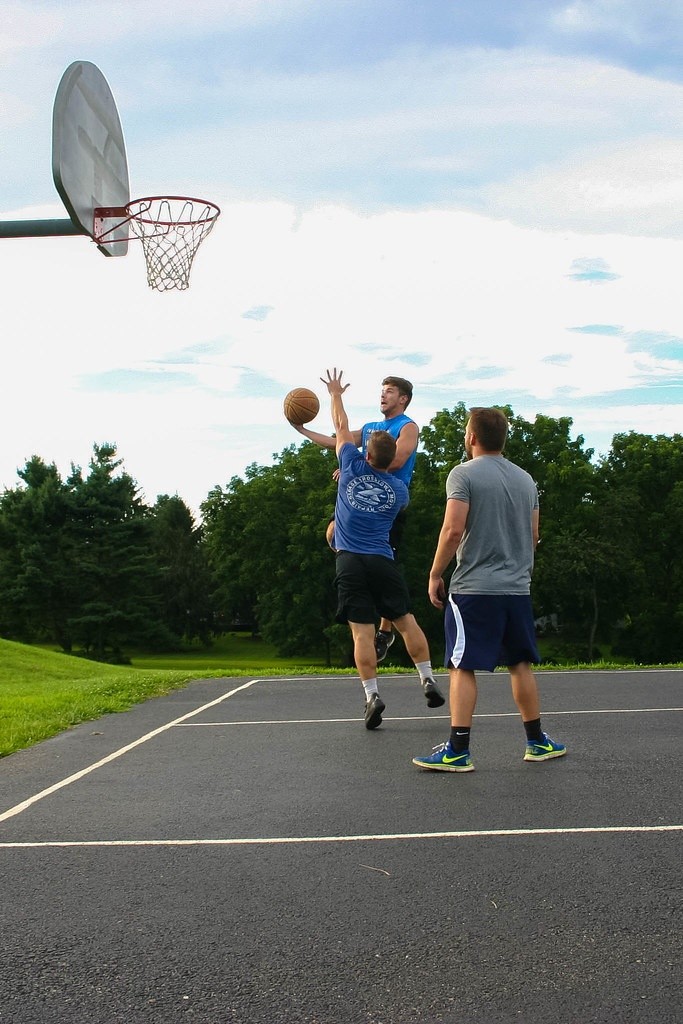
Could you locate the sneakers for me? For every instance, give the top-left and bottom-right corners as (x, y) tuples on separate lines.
(375, 632), (396, 662)
(423, 677), (445, 708)
(521, 733), (568, 760)
(363, 692), (385, 728)
(413, 742), (476, 772)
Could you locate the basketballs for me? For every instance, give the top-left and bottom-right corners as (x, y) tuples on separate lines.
(284, 387), (320, 425)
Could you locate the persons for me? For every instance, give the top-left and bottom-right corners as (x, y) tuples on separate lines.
(319, 367), (445, 731)
(284, 377), (420, 663)
(410, 407), (566, 772)
